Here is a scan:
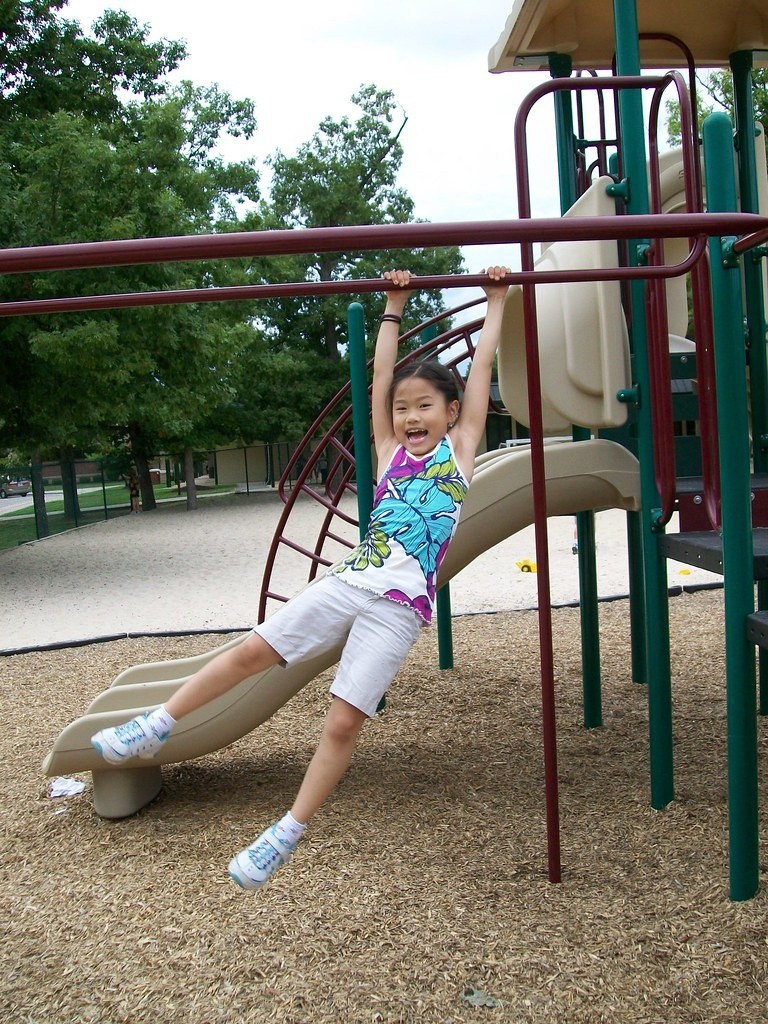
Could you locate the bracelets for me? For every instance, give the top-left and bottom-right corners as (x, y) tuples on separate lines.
(381, 314), (401, 319)
(380, 319), (401, 325)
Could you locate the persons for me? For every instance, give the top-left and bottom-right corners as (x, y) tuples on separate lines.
(91, 263), (518, 889)
(129, 477), (140, 515)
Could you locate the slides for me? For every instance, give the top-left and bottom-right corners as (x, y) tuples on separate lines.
(38, 437), (643, 779)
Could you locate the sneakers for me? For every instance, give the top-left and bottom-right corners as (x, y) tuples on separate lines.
(91, 713), (169, 764)
(227, 822), (297, 890)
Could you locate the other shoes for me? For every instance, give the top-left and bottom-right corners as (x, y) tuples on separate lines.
(130, 511), (135, 514)
(137, 510), (140, 513)
